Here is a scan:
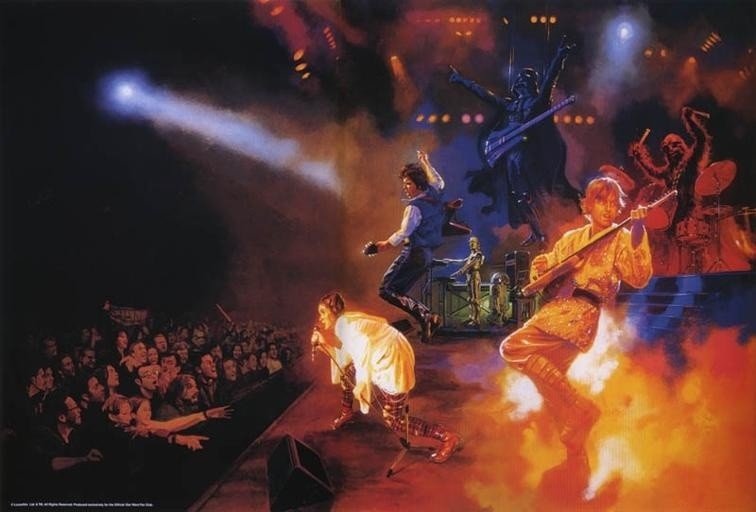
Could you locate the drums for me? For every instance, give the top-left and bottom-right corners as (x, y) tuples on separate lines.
(647, 235), (692, 276)
(676, 217), (712, 245)
(635, 183), (679, 231)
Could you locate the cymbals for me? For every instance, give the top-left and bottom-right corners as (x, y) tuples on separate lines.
(703, 204), (732, 215)
(600, 165), (635, 194)
(734, 208), (755, 216)
(695, 160), (736, 197)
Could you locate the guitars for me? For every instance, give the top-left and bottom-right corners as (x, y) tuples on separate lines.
(521, 190), (676, 298)
(484, 94), (576, 168)
(362, 198), (471, 257)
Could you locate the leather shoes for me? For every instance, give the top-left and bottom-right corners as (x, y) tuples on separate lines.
(420, 313), (442, 341)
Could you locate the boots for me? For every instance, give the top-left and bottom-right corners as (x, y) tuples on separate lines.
(427, 429), (466, 464)
(521, 351), (600, 488)
(331, 409), (361, 430)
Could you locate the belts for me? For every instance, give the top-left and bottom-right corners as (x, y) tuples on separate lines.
(555, 283), (602, 307)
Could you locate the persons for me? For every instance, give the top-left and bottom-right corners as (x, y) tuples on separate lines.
(446, 236), (482, 325)
(309, 289), (466, 464)
(495, 176), (655, 482)
(447, 37), (583, 249)
(374, 150), (448, 345)
